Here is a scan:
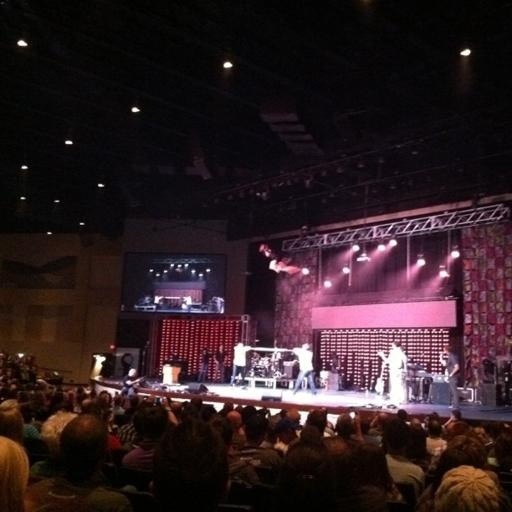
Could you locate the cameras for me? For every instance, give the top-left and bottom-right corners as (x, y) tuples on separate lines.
(154, 397), (162, 404)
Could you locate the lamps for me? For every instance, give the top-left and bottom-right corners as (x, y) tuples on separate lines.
(343, 235), (461, 278)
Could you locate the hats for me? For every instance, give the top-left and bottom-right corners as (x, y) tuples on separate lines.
(433, 465), (501, 512)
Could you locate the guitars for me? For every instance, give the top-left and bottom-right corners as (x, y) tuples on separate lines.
(375, 361), (386, 394)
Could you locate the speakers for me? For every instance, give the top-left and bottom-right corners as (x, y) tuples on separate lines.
(284, 366), (296, 379)
(189, 383), (208, 394)
(430, 382), (451, 405)
(262, 393), (282, 401)
(477, 382), (502, 407)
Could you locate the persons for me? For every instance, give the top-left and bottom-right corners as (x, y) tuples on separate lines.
(0, 341), (512, 512)
(151, 294), (224, 313)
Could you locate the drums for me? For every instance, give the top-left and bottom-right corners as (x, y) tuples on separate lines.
(250, 364), (268, 378)
